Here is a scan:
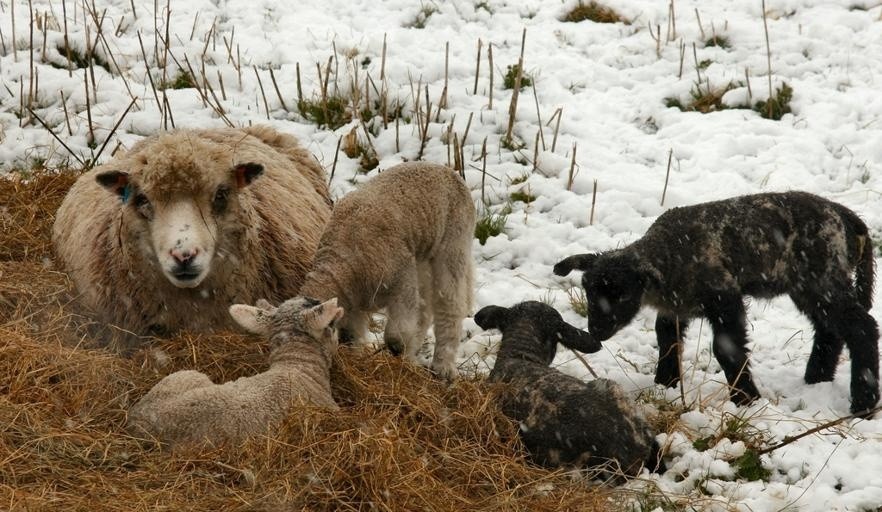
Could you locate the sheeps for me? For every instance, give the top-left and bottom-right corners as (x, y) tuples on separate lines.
(52, 124), (332, 337)
(473, 299), (665, 487)
(552, 191), (881, 420)
(127, 294), (339, 453)
(255, 160), (476, 381)
